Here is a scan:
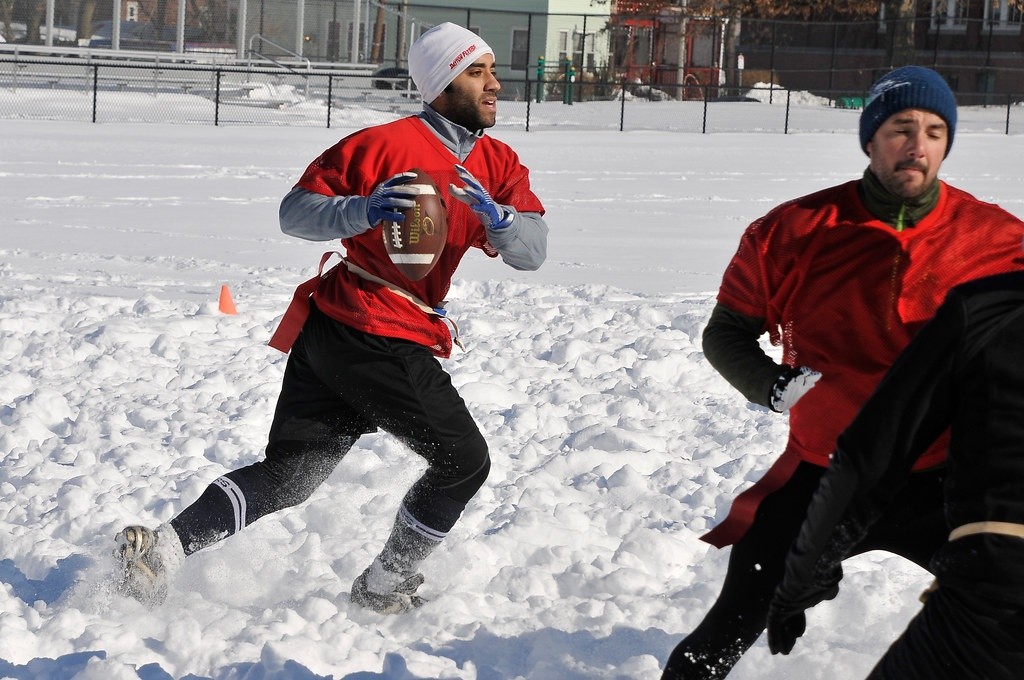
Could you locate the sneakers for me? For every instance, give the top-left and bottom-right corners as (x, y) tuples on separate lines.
(350, 569), (431, 615)
(113, 525), (166, 609)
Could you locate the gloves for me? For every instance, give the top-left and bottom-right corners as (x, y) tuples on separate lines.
(767, 582), (829, 656)
(448, 164), (514, 231)
(366, 171), (419, 228)
(769, 365), (822, 414)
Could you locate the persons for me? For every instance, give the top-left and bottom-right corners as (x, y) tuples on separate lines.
(112, 23), (548, 615)
(658, 67), (1024, 680)
(766, 271), (1023, 680)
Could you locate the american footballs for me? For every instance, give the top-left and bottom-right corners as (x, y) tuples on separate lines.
(381, 166), (449, 283)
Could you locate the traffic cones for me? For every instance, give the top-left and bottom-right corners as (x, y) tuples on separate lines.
(218, 284), (238, 315)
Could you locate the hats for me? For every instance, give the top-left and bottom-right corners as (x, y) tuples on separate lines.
(859, 65), (957, 159)
(408, 22), (495, 106)
